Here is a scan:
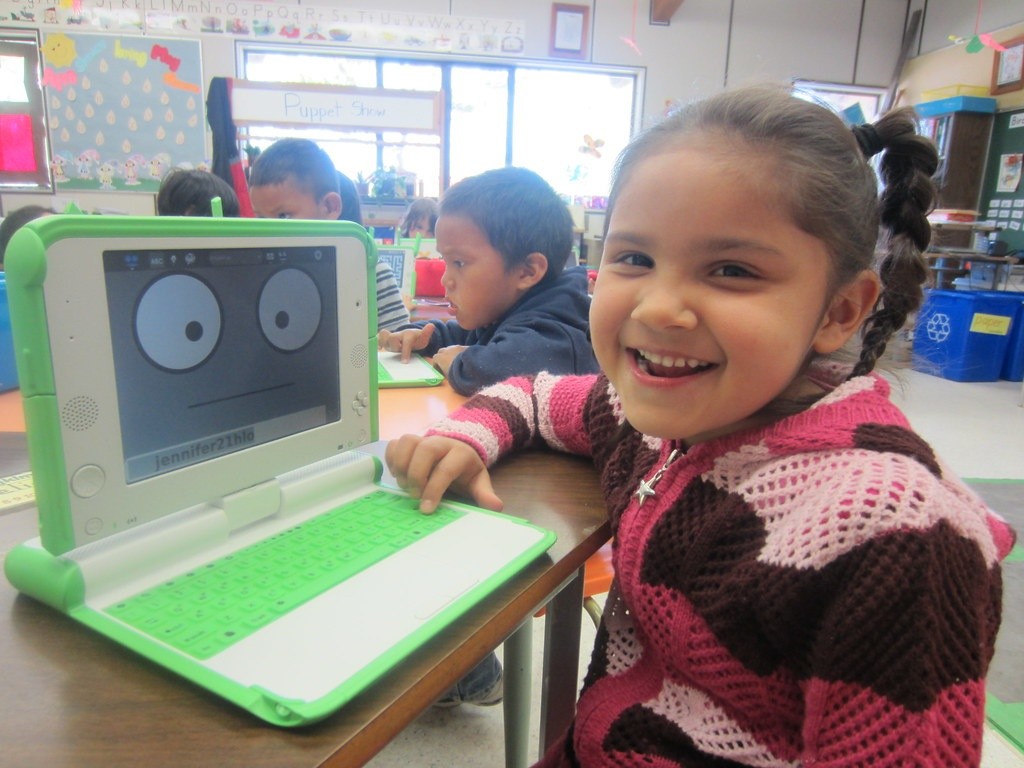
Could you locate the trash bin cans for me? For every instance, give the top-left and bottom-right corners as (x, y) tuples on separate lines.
(907, 288), (1024, 382)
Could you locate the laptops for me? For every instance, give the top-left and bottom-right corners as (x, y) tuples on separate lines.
(366, 226), (459, 389)
(3, 213), (557, 734)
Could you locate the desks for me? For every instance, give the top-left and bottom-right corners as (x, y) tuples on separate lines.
(0, 353), (611, 768)
(923, 254), (1022, 291)
(928, 223), (997, 252)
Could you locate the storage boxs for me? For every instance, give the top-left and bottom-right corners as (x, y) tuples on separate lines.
(910, 291), (1024, 383)
(927, 208), (983, 223)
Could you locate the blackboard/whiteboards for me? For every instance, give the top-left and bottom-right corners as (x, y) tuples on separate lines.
(956, 109), (1024, 261)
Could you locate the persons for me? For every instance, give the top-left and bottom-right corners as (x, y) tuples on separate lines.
(383, 87), (1016, 768)
(245, 138), (411, 349)
(157, 169), (240, 220)
(0, 205), (60, 268)
(377, 166), (603, 397)
